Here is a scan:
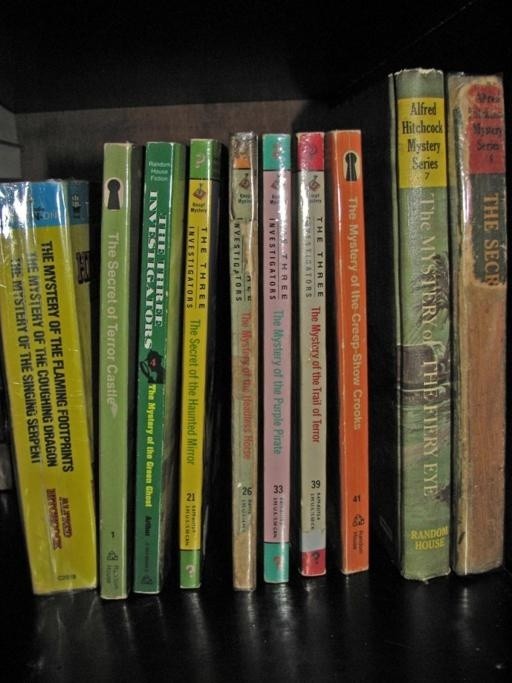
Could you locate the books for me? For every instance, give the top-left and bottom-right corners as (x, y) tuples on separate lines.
(329, 129), (371, 576)
(134, 140), (188, 594)
(385, 67), (452, 582)
(1, 178), (98, 596)
(228, 130), (259, 593)
(296, 131), (329, 576)
(100, 140), (144, 601)
(446, 70), (508, 576)
(263, 132), (294, 584)
(179, 137), (230, 589)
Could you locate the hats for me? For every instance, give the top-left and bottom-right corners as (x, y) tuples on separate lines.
(145, 348), (164, 361)
